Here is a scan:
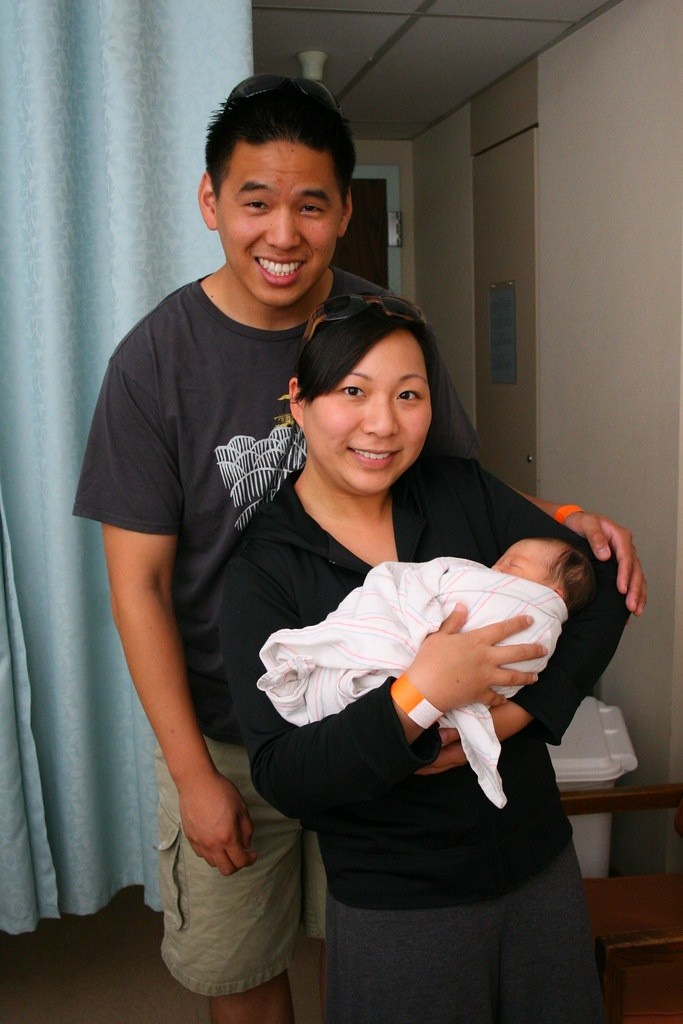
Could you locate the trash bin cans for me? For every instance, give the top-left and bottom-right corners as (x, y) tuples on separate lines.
(550, 699), (640, 880)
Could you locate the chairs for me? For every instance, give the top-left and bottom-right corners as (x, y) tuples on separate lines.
(558, 784), (683, 1024)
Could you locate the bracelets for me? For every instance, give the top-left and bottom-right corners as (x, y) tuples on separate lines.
(552, 505), (585, 524)
(390, 673), (445, 729)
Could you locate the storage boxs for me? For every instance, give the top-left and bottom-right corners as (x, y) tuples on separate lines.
(545, 695), (638, 880)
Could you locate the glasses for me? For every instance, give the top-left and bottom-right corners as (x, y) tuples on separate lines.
(222, 73), (346, 124)
(296, 293), (430, 381)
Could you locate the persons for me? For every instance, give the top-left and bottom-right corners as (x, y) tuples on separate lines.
(216, 292), (631, 1023)
(256, 537), (594, 729)
(70, 78), (649, 1024)
